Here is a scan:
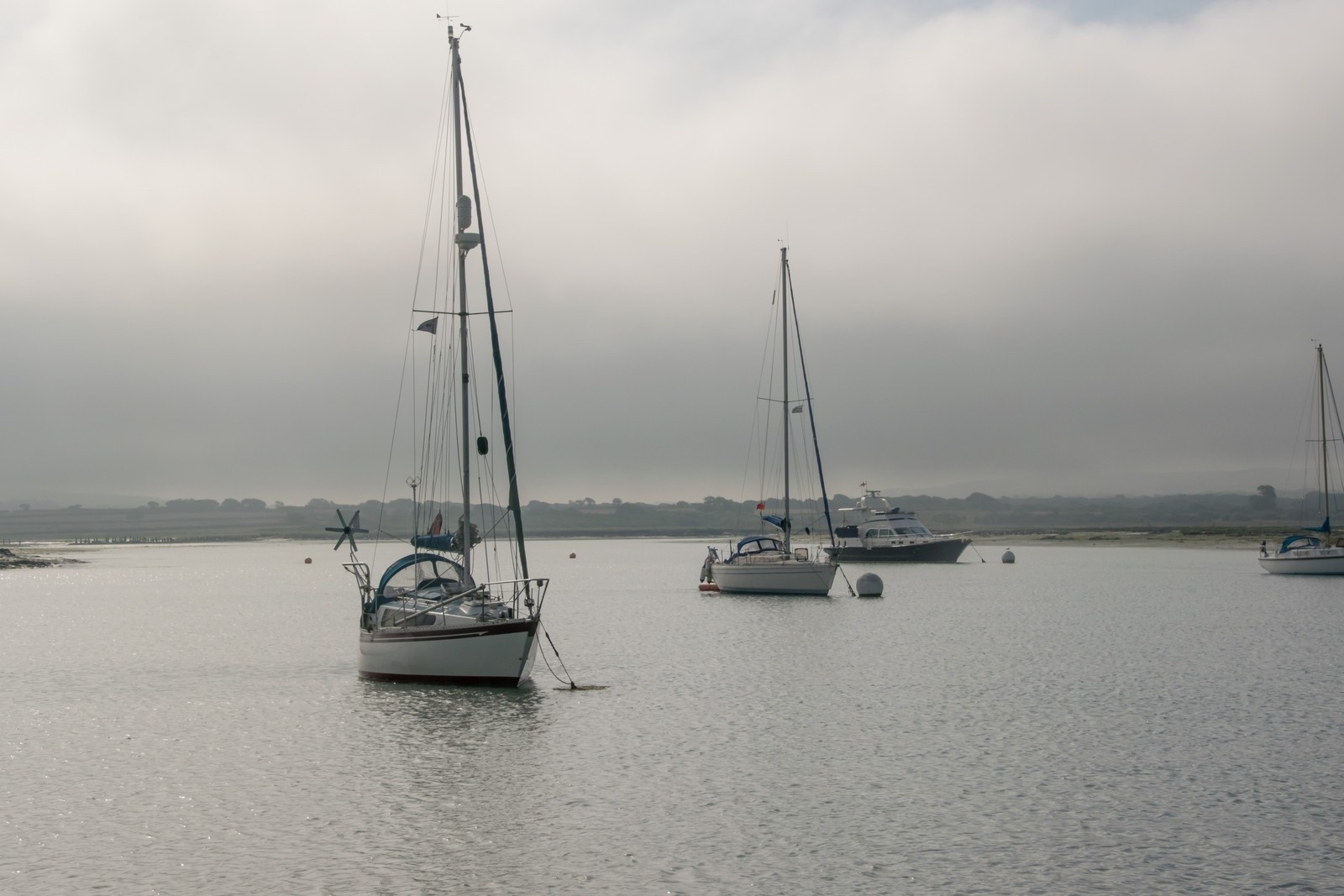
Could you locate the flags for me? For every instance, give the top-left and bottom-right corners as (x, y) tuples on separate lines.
(756, 502), (764, 509)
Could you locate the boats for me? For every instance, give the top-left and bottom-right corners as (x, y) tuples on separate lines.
(814, 474), (977, 564)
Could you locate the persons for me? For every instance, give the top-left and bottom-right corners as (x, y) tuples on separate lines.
(1261, 541), (1267, 557)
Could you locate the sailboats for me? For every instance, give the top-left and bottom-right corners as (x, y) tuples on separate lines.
(693, 245), (845, 595)
(1256, 337), (1343, 577)
(325, 13), (555, 688)
